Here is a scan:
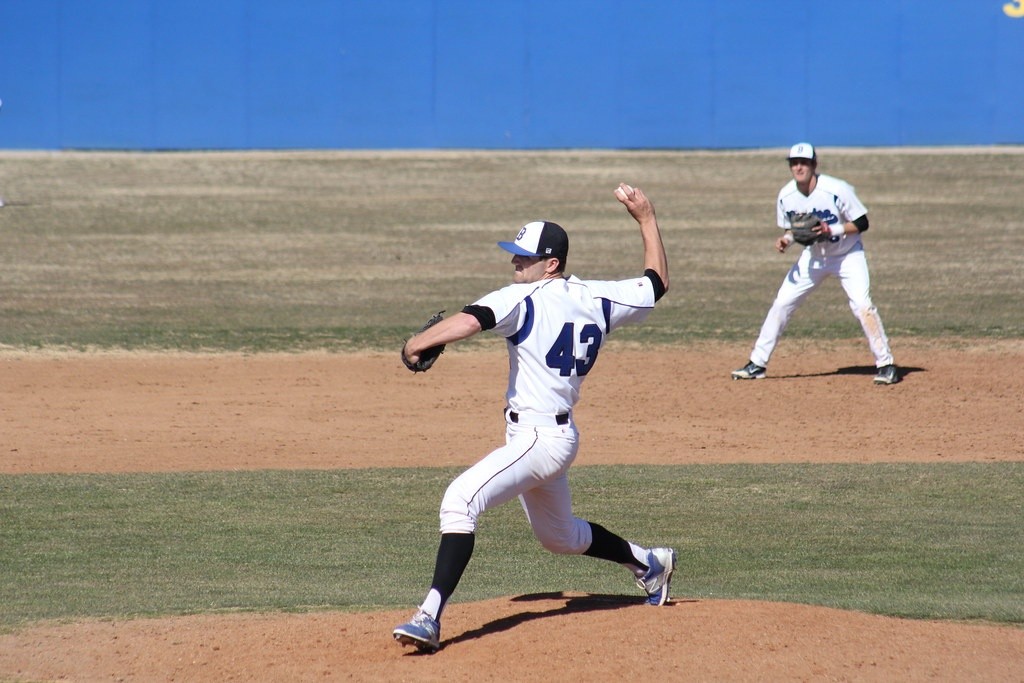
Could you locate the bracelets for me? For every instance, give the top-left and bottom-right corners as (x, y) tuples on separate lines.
(783, 234), (794, 243)
(829, 224), (845, 237)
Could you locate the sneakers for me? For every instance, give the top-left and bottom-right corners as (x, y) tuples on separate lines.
(635, 547), (677, 607)
(730, 361), (766, 380)
(393, 604), (440, 652)
(873, 364), (899, 385)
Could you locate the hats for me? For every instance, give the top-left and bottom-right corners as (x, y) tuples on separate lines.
(497, 221), (569, 257)
(786, 142), (816, 161)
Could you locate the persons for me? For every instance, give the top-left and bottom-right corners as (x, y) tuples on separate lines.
(392, 183), (675, 649)
(731, 143), (901, 384)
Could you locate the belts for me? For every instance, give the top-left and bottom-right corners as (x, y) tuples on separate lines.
(504, 408), (568, 424)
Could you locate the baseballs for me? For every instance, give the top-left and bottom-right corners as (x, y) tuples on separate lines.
(618, 185), (634, 200)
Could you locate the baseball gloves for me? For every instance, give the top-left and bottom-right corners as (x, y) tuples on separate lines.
(400, 309), (446, 375)
(791, 212), (821, 246)
